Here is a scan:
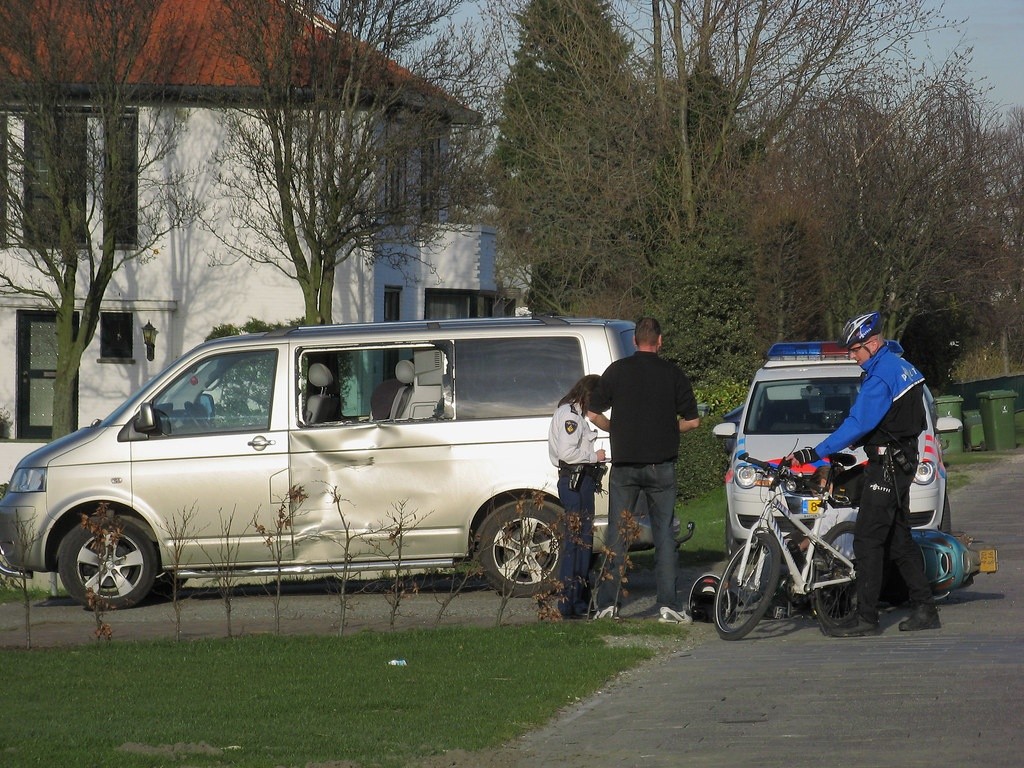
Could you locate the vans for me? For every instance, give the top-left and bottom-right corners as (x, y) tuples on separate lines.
(1, 306), (695, 613)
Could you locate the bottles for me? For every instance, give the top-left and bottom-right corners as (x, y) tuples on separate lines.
(787, 539), (806, 568)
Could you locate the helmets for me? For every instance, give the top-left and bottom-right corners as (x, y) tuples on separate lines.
(837, 311), (882, 348)
(688, 574), (731, 621)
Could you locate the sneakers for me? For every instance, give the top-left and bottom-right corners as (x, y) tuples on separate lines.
(899, 604), (941, 631)
(659, 607), (693, 625)
(829, 612), (880, 637)
(592, 604), (618, 620)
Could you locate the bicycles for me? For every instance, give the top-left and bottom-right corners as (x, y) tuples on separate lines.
(714, 453), (866, 641)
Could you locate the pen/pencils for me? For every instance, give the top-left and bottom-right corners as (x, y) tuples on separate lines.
(601, 441), (603, 450)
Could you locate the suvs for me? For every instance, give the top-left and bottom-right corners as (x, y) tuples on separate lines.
(720, 340), (964, 552)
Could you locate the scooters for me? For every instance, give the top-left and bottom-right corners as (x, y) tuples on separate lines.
(729, 527), (1000, 623)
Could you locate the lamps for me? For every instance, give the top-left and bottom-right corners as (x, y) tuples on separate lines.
(141, 320), (156, 350)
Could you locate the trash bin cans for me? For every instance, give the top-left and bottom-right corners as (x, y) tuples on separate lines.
(934, 394), (964, 457)
(961, 409), (986, 452)
(976, 389), (1019, 451)
(723, 403), (745, 455)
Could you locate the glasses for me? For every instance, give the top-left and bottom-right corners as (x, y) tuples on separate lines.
(848, 340), (873, 354)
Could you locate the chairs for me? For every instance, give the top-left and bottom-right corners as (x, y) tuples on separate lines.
(304, 358), (415, 423)
(759, 402), (808, 436)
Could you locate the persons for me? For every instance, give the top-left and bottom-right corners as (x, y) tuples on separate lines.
(589, 317), (699, 625)
(786, 313), (941, 637)
(549, 375), (606, 619)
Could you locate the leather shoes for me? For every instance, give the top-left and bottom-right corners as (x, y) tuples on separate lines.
(562, 609), (597, 620)
